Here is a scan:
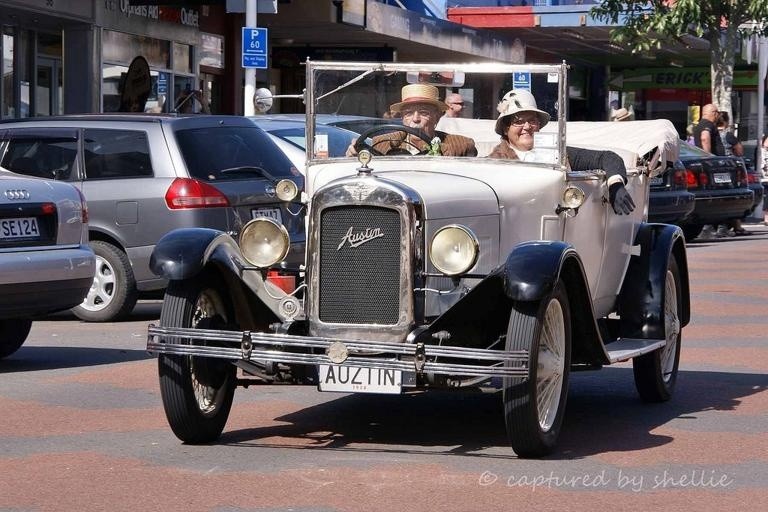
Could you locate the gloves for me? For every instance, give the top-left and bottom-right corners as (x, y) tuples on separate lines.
(607, 181), (638, 218)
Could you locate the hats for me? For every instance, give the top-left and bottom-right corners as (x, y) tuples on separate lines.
(613, 107), (634, 122)
(388, 82), (451, 114)
(494, 88), (552, 137)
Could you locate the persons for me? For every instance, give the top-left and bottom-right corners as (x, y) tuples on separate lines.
(476, 89), (636, 216)
(346, 85), (478, 157)
(179, 93), (211, 114)
(614, 108), (633, 121)
(443, 93), (467, 117)
(128, 94), (142, 112)
(692, 104), (768, 237)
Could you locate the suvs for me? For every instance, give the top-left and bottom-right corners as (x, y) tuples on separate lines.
(145, 56), (692, 457)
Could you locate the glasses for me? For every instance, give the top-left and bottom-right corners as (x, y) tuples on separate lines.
(509, 115), (541, 128)
(400, 108), (439, 118)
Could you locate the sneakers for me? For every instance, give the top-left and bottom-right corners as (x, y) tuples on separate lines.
(697, 224), (754, 239)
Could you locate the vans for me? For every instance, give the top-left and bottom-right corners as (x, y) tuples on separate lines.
(0, 112), (309, 321)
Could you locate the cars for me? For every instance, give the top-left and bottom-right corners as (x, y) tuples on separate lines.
(645, 155), (694, 224)
(1, 167), (95, 360)
(741, 157), (762, 218)
(242, 115), (405, 140)
(245, 115), (378, 178)
(676, 138), (754, 244)
